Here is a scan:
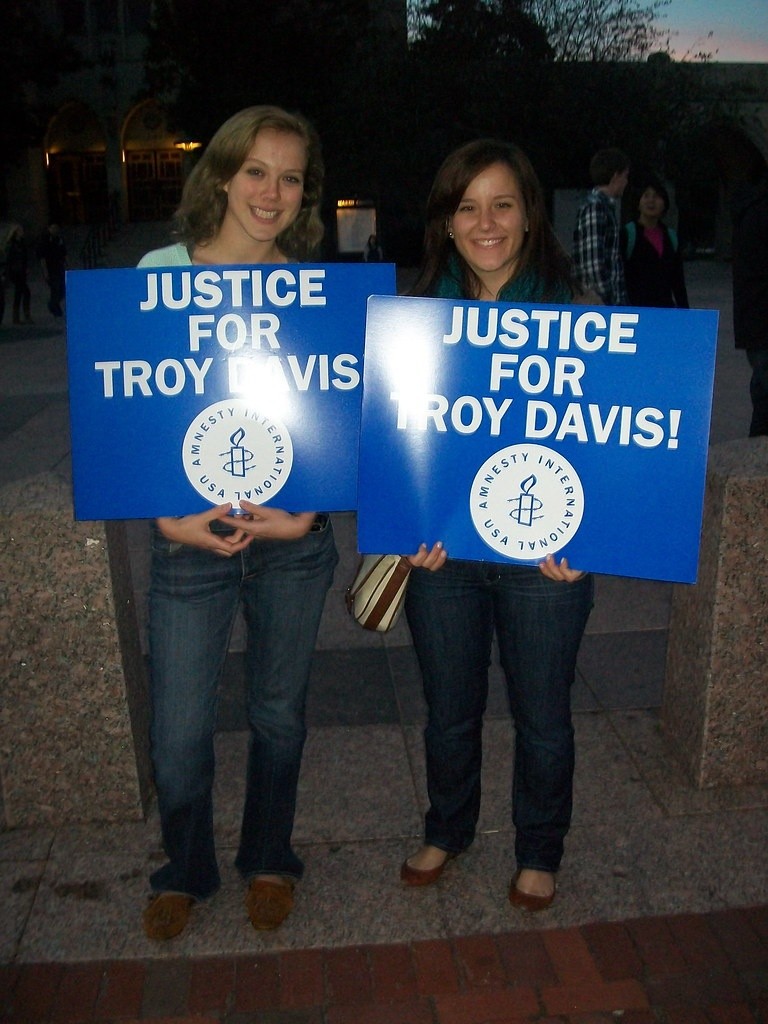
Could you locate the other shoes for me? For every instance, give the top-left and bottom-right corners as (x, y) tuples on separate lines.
(144, 891), (192, 939)
(245, 877), (293, 929)
(510, 866), (555, 912)
(401, 854), (449, 886)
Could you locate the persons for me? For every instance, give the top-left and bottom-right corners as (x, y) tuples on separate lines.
(730, 178), (768, 436)
(569, 149), (629, 306)
(137, 106), (339, 940)
(617, 181), (689, 307)
(37, 222), (71, 317)
(5, 224), (35, 326)
(400, 137), (594, 912)
(363, 235), (383, 262)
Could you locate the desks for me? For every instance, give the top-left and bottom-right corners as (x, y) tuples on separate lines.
(337, 206), (384, 263)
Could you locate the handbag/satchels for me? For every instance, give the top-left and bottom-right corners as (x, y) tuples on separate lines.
(346, 552), (412, 632)
